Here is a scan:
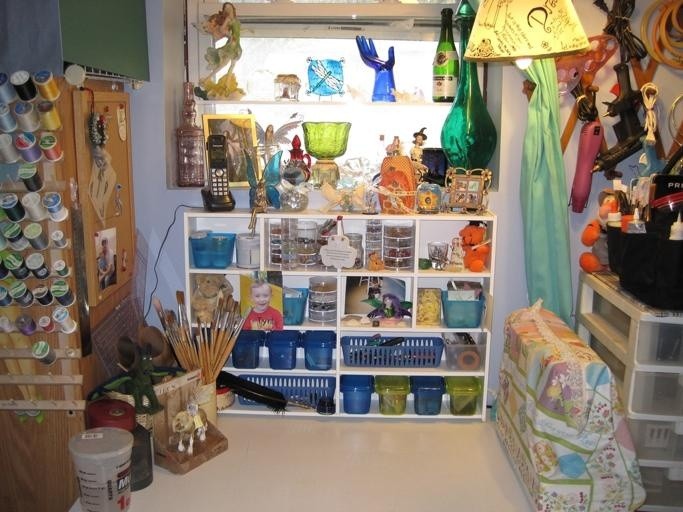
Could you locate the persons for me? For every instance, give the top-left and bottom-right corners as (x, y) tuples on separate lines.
(204, 2), (242, 89)
(356, 35), (396, 101)
(242, 282), (283, 333)
(411, 125), (429, 182)
(98, 238), (115, 289)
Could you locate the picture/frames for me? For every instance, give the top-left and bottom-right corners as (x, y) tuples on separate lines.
(441, 166), (492, 214)
(74, 89), (136, 308)
(204, 114), (262, 188)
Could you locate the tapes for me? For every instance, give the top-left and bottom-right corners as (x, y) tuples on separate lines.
(609, 212), (621, 221)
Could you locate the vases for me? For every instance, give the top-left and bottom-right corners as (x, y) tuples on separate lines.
(302, 121), (350, 189)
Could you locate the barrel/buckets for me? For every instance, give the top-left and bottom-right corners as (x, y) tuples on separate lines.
(68, 427), (132, 512)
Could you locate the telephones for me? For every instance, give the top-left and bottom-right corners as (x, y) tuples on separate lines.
(201, 135), (235, 210)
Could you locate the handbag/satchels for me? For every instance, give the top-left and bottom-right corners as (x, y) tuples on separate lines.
(604, 206), (683, 311)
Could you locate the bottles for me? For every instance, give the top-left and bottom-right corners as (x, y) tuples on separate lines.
(342, 233), (364, 269)
(177, 81), (206, 187)
(432, 9), (458, 102)
(306, 276), (339, 324)
(450, 237), (462, 269)
(273, 74), (301, 105)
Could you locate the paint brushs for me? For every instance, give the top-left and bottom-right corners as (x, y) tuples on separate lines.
(153, 288), (253, 386)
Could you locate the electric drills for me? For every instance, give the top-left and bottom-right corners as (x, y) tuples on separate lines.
(589, 63), (649, 175)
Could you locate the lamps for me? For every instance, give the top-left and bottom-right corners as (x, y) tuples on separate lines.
(463, 0), (592, 70)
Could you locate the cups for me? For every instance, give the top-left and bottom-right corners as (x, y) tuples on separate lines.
(428, 241), (448, 270)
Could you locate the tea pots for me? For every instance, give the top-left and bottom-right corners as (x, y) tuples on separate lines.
(282, 135), (312, 185)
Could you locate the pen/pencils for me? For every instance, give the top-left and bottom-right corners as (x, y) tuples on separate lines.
(612, 177), (651, 233)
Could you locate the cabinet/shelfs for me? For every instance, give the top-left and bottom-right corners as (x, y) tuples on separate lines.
(575, 270), (683, 512)
(183, 208), (497, 422)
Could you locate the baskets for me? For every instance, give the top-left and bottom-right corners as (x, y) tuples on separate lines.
(238, 375), (335, 407)
(341, 335), (445, 367)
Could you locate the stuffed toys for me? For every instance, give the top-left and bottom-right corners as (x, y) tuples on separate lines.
(192, 274), (233, 324)
(579, 190), (633, 273)
(129, 344), (164, 414)
(459, 221), (491, 272)
